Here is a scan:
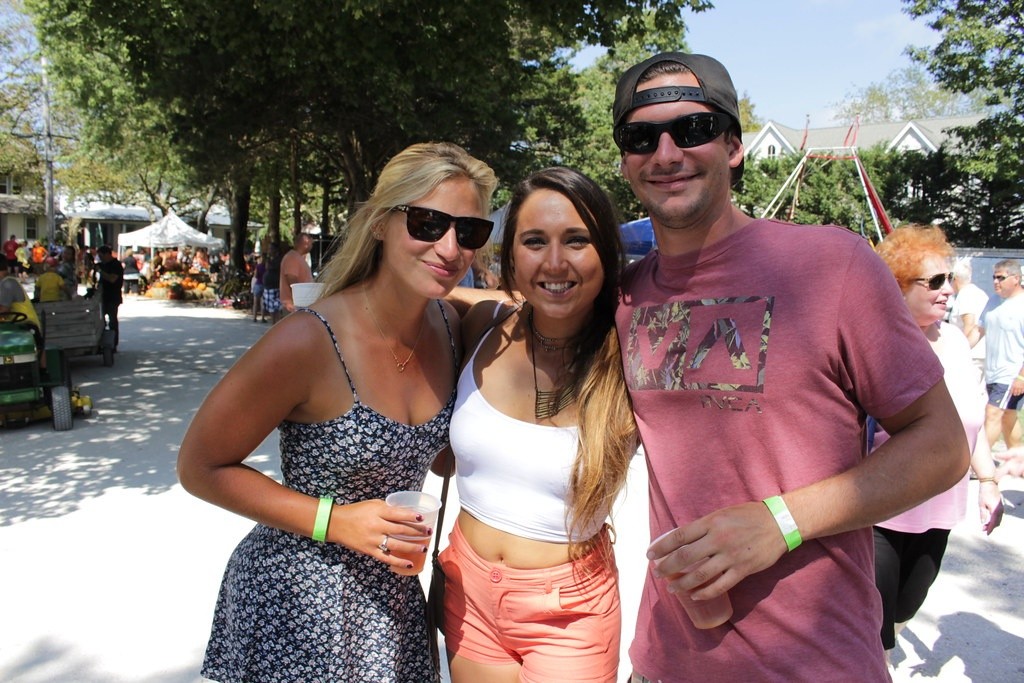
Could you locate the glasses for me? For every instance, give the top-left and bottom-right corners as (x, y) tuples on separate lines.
(394, 204), (494, 249)
(993, 275), (1011, 281)
(618, 113), (731, 154)
(909, 272), (955, 291)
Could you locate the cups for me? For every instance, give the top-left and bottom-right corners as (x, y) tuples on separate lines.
(649, 527), (733, 628)
(290, 283), (325, 311)
(384, 491), (443, 575)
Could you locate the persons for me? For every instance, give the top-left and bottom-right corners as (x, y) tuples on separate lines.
(250, 232), (314, 321)
(435, 167), (641, 683)
(0, 254), (46, 372)
(946, 259), (1024, 477)
(109, 245), (260, 293)
(458, 254), (503, 289)
(177, 140), (499, 683)
(445, 53), (971, 683)
(864, 224), (1005, 657)
(3, 235), (100, 302)
(91, 246), (123, 351)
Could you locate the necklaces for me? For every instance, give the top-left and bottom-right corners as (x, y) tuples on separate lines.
(361, 286), (425, 372)
(531, 307), (576, 420)
(528, 312), (573, 351)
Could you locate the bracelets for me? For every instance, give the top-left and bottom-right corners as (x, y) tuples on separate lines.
(1017, 375), (1024, 381)
(764, 496), (801, 552)
(312, 498), (334, 542)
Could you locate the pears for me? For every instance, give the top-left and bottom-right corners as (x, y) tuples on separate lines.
(70, 395), (93, 406)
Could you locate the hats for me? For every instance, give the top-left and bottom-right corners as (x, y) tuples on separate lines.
(614, 51), (745, 184)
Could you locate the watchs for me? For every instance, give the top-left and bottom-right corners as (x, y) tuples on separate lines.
(979, 472), (999, 484)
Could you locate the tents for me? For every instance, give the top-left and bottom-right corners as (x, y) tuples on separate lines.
(619, 217), (657, 261)
(117, 212), (225, 261)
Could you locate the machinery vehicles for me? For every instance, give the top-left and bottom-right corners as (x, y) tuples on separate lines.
(0, 289), (116, 430)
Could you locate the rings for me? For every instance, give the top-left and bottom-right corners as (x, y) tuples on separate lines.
(379, 536), (388, 552)
